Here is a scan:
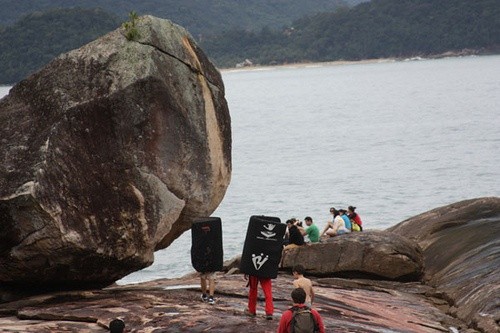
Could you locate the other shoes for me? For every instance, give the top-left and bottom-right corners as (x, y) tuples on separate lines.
(201, 294), (207, 302)
(262, 313), (273, 320)
(208, 298), (214, 304)
(244, 308), (256, 318)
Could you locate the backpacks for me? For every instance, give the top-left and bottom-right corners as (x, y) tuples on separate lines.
(288, 306), (319, 333)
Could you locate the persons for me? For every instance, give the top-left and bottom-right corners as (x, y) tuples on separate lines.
(277, 220), (306, 267)
(278, 288), (325, 333)
(289, 265), (315, 307)
(242, 273), (274, 320)
(290, 218), (306, 235)
(320, 205), (363, 237)
(302, 217), (321, 242)
(198, 272), (217, 304)
